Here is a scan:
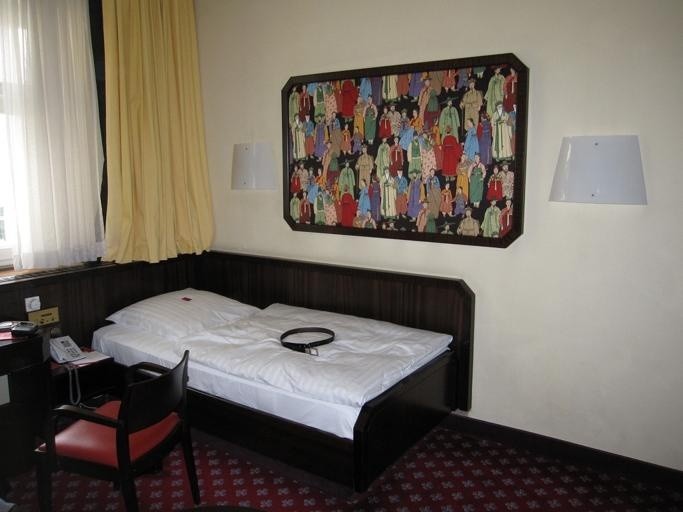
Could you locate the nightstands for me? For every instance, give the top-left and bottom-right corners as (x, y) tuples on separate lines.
(44, 346), (114, 407)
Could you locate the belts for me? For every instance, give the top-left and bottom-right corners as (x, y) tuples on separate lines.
(280, 327), (335, 356)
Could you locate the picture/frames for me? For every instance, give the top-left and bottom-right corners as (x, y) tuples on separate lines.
(279, 51), (528, 248)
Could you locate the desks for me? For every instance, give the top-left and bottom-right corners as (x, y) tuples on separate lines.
(0, 331), (51, 511)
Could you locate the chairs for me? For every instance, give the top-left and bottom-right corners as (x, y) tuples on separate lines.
(34, 349), (202, 512)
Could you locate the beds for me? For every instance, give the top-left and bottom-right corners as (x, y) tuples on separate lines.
(94, 302), (457, 495)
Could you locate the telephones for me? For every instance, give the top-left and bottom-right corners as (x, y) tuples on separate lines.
(49, 335), (86, 363)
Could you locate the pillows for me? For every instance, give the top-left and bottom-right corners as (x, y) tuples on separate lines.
(103, 286), (262, 341)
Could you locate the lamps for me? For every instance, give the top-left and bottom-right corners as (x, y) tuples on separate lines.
(231, 143), (277, 191)
(549, 135), (650, 207)
